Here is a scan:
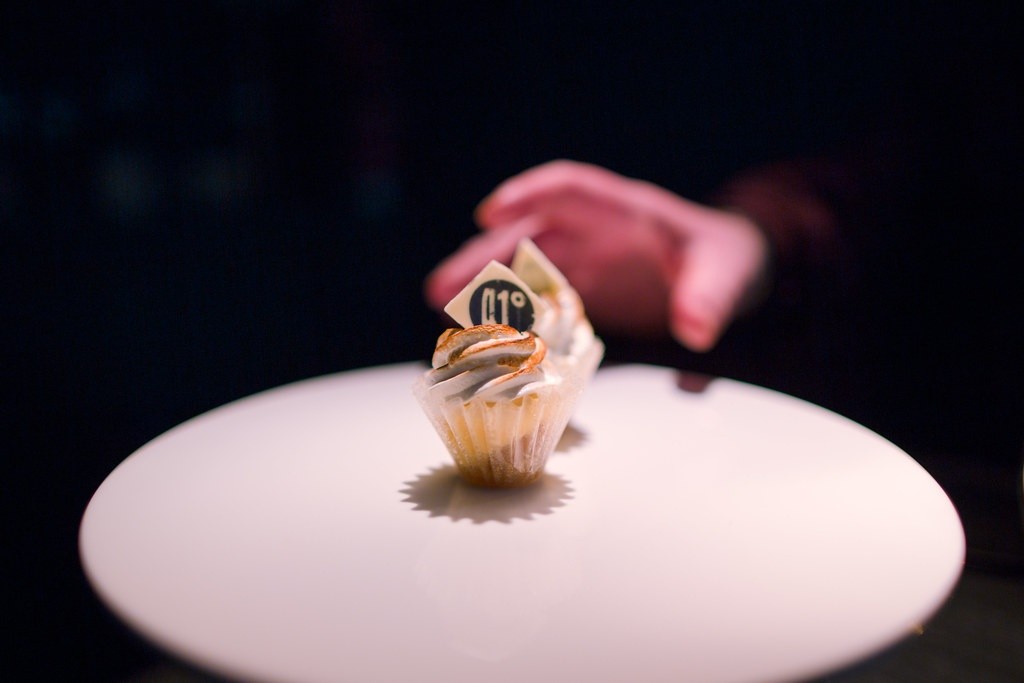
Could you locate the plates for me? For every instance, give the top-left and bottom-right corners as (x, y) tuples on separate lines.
(79, 360), (965, 683)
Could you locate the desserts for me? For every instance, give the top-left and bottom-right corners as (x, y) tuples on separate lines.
(415, 236), (606, 490)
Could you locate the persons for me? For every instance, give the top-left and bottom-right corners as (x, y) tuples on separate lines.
(428, 159), (836, 369)
